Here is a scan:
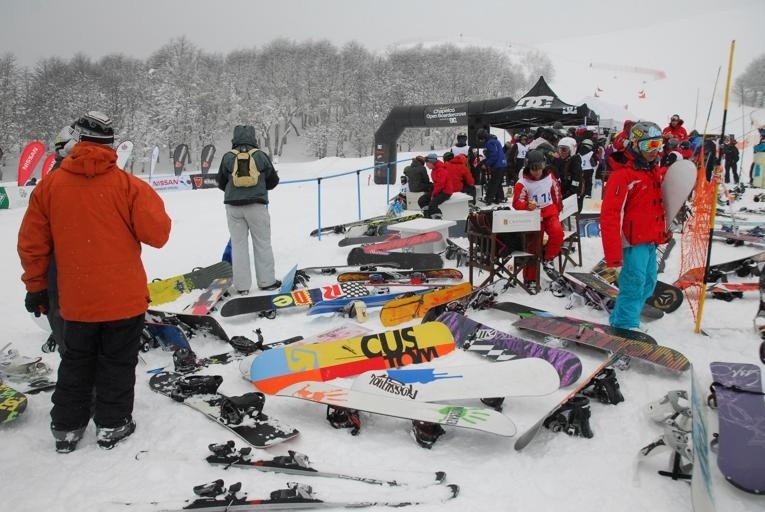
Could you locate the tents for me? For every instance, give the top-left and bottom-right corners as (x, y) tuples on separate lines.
(489, 74), (599, 130)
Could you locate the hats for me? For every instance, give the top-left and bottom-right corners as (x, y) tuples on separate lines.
(670, 114), (681, 122)
(54, 126), (72, 153)
(476, 129), (489, 140)
(428, 153), (438, 163)
(668, 138), (690, 149)
(67, 111), (115, 149)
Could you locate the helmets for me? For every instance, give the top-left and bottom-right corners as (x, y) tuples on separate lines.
(558, 136), (578, 158)
(628, 121), (663, 145)
(581, 139), (594, 151)
(523, 149), (545, 168)
(416, 156), (425, 164)
(457, 132), (467, 139)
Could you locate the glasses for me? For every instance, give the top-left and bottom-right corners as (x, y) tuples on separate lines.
(529, 160), (547, 171)
(424, 157), (428, 162)
(556, 146), (569, 154)
(638, 137), (664, 153)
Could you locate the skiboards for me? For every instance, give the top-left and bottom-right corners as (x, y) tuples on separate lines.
(310, 214), (425, 236)
(114, 443), (459, 512)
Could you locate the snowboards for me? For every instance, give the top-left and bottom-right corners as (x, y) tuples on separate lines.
(379, 282), (474, 326)
(149, 369), (300, 449)
(147, 259), (232, 307)
(350, 355), (563, 411)
(660, 158), (697, 235)
(179, 276), (233, 318)
(141, 328), (304, 377)
(593, 267), (681, 315)
(515, 339), (632, 450)
(540, 260), (623, 313)
(434, 310), (583, 388)
(275, 379), (517, 449)
(337, 268), (463, 287)
(708, 362), (764, 496)
(308, 289), (436, 318)
(251, 320), (456, 397)
(493, 301), (660, 349)
(220, 281), (370, 318)
(511, 315), (691, 376)
(239, 324), (374, 384)
(673, 207), (765, 301)
(275, 232), (449, 295)
(137, 308), (231, 351)
(0, 342), (56, 424)
(641, 360), (716, 512)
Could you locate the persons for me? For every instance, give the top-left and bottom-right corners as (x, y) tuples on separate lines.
(16, 108), (171, 454)
(216, 123), (282, 295)
(511, 146), (564, 297)
(44, 122), (77, 352)
(602, 122), (677, 335)
(401, 114), (740, 221)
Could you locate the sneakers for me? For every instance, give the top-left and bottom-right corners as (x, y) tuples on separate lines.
(543, 259), (555, 274)
(96, 419), (137, 450)
(260, 279), (283, 291)
(524, 281), (542, 291)
(55, 436), (82, 454)
(237, 289), (250, 295)
(478, 195), (503, 204)
(629, 323), (650, 335)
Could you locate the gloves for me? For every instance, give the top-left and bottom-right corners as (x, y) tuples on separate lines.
(24, 289), (49, 317)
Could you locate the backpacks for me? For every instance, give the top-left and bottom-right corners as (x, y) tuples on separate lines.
(228, 147), (262, 189)
